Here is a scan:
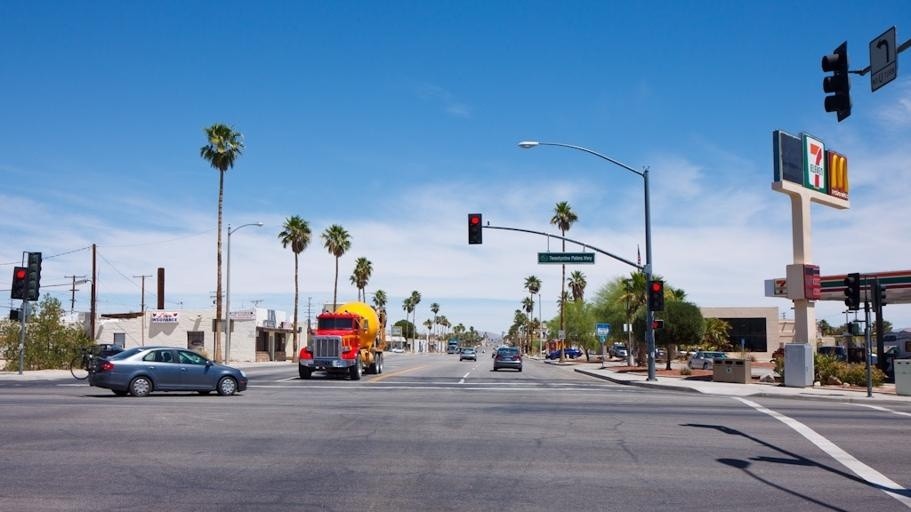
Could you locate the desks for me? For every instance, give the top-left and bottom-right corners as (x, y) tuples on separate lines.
(521, 290), (543, 358)
(822, 40), (851, 123)
(518, 141), (659, 382)
(225, 222), (264, 365)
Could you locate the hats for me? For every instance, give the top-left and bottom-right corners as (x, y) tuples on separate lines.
(459, 348), (478, 361)
(542, 350), (547, 354)
(687, 351), (736, 369)
(492, 347), (524, 372)
(636, 344), (668, 362)
(545, 348), (583, 360)
(609, 341), (634, 359)
(390, 347), (406, 353)
(87, 343), (127, 358)
(89, 346), (248, 397)
(492, 345), (510, 359)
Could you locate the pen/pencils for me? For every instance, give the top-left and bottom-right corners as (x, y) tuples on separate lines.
(817, 346), (848, 361)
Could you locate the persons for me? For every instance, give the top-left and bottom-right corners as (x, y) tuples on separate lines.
(163, 353), (172, 360)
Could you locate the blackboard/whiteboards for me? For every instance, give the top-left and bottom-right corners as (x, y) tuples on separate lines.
(299, 301), (384, 380)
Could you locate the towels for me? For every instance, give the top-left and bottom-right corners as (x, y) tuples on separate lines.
(713, 358), (752, 384)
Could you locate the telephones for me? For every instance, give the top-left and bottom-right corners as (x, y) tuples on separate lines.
(539, 252), (595, 264)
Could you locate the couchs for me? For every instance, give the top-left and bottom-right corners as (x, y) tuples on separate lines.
(844, 272), (861, 310)
(877, 279), (888, 313)
(654, 320), (664, 329)
(650, 280), (665, 311)
(846, 322), (861, 334)
(468, 214), (483, 245)
(11, 266), (28, 300)
